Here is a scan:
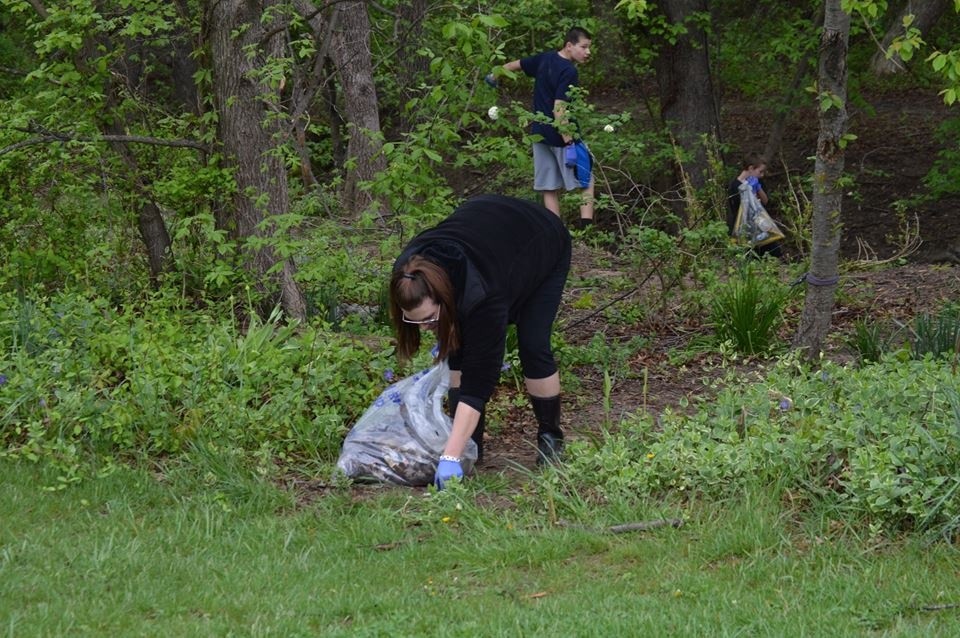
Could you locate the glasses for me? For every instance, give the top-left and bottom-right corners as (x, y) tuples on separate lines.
(401, 304), (441, 324)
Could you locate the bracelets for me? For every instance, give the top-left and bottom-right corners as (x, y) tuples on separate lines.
(440, 455), (461, 463)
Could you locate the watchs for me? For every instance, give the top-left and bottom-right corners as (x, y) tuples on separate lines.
(564, 139), (575, 146)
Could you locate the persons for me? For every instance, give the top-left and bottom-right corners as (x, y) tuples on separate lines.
(388, 196), (572, 492)
(729, 151), (783, 259)
(486, 26), (596, 229)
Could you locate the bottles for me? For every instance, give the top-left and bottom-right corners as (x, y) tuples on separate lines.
(566, 141), (577, 168)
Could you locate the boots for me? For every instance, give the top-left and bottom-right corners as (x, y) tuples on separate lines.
(526, 392), (564, 467)
(470, 401), (487, 465)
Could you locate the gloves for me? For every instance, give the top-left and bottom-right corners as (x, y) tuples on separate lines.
(486, 74), (499, 90)
(564, 142), (577, 169)
(747, 175), (760, 193)
(434, 460), (463, 493)
